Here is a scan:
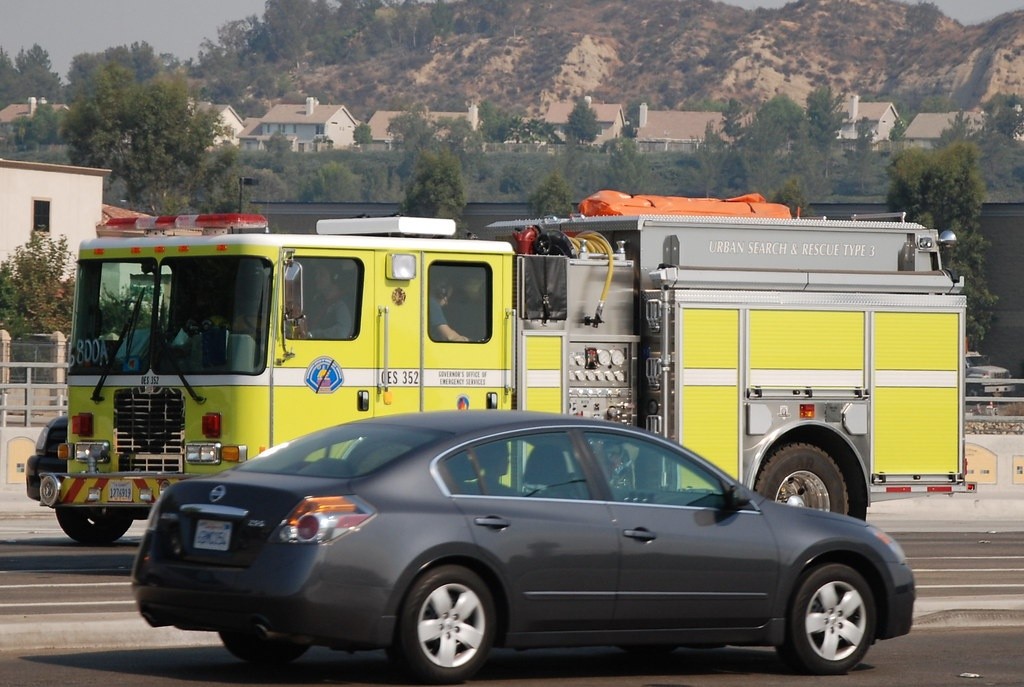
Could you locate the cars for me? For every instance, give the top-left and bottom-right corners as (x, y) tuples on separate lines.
(130, 409), (917, 687)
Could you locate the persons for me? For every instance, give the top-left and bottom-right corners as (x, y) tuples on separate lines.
(429, 296), (472, 341)
(484, 444), (510, 483)
(306, 265), (352, 338)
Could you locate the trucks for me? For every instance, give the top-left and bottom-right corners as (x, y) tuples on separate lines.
(964, 349), (1016, 406)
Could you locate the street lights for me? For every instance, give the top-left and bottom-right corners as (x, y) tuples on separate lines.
(237, 177), (259, 234)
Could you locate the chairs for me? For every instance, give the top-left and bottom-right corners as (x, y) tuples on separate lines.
(453, 452), (504, 496)
(525, 445), (585, 500)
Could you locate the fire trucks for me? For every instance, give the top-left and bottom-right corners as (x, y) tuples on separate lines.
(26, 188), (979, 550)
